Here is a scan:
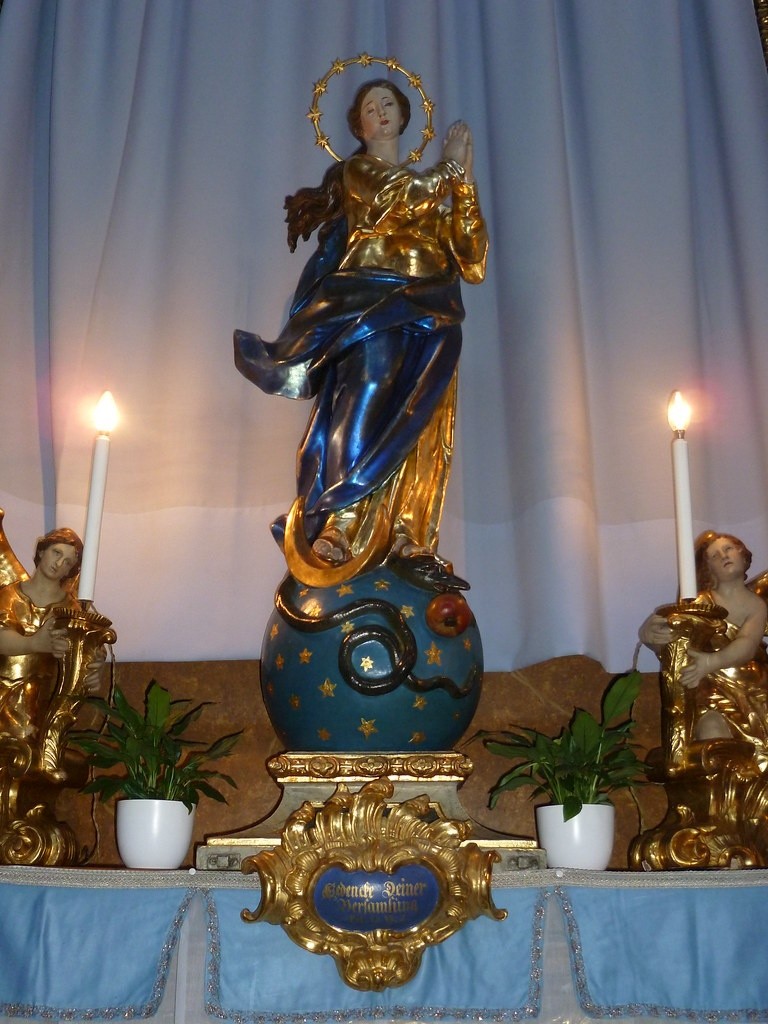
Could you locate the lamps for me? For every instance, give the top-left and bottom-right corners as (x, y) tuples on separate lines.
(79, 390), (123, 601)
(667, 390), (699, 603)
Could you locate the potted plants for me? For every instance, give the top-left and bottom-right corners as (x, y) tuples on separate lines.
(458, 669), (654, 871)
(62, 678), (247, 870)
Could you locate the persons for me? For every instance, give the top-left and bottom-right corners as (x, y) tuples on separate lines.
(0, 528), (109, 865)
(636, 532), (768, 739)
(233, 78), (487, 566)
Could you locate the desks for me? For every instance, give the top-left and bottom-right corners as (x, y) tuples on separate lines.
(1, 864), (768, 1023)
